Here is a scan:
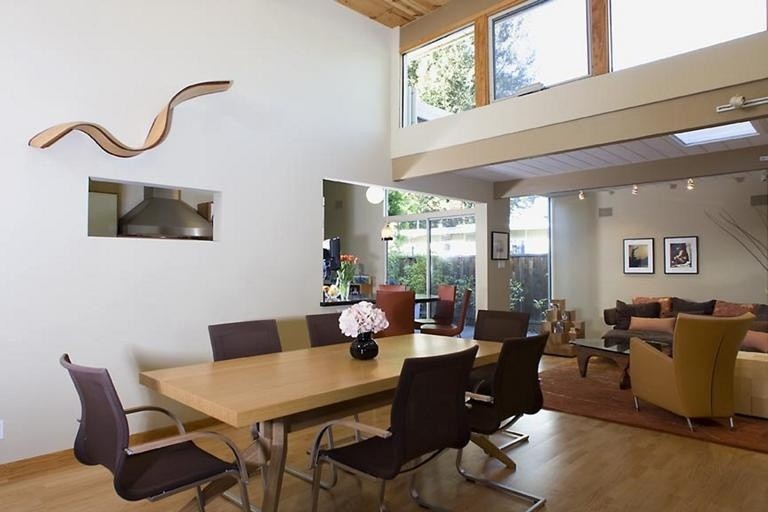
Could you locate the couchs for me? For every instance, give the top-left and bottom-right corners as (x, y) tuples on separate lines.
(601, 299), (767, 419)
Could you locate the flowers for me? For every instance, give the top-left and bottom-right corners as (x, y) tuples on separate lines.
(337, 298), (390, 340)
(335, 255), (360, 282)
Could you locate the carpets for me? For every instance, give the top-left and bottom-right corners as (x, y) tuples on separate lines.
(538, 356), (767, 455)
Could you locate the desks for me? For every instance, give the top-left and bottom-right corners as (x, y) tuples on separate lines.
(138, 329), (502, 512)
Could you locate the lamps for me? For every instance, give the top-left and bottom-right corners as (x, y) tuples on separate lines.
(574, 176), (696, 202)
(729, 96), (746, 109)
(381, 223), (394, 242)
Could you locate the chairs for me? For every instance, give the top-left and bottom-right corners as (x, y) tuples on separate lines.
(472, 306), (532, 454)
(308, 311), (345, 346)
(58, 352), (252, 512)
(206, 317), (282, 363)
(408, 331), (552, 512)
(630, 313), (755, 434)
(309, 345), (479, 511)
(345, 282), (475, 337)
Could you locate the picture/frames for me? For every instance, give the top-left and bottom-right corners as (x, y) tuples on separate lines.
(622, 238), (655, 275)
(663, 235), (699, 275)
(490, 230), (510, 261)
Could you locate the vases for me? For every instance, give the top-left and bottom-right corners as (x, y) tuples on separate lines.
(349, 330), (382, 360)
(340, 281), (350, 302)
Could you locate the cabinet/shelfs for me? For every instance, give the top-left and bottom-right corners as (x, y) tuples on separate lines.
(539, 298), (585, 357)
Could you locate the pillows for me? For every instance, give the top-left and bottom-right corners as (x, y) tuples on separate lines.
(611, 296), (768, 350)
